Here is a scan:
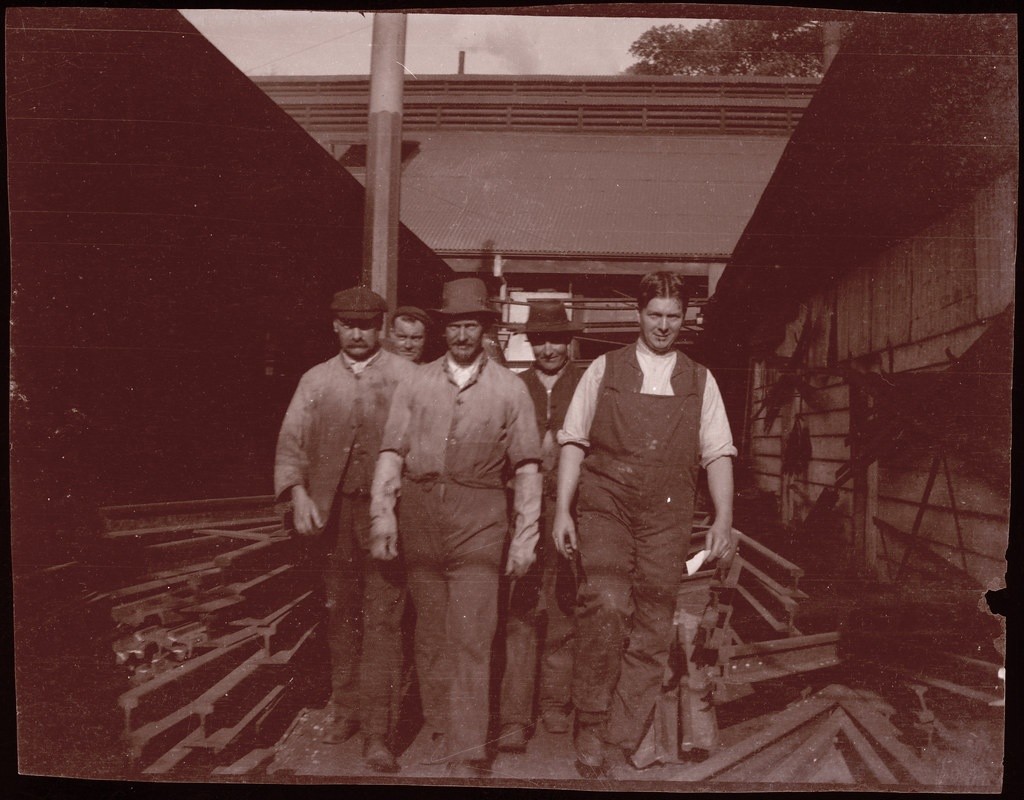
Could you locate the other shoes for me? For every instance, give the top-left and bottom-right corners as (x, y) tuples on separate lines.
(572, 721), (602, 768)
(363, 735), (401, 772)
(463, 741), (495, 773)
(603, 745), (627, 776)
(323, 716), (359, 745)
(497, 724), (529, 750)
(419, 735), (449, 765)
(542, 711), (569, 732)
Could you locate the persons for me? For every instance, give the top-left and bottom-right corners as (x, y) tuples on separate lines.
(367, 279), (543, 765)
(389, 305), (433, 365)
(273, 285), (423, 773)
(553, 270), (738, 778)
(495, 299), (589, 752)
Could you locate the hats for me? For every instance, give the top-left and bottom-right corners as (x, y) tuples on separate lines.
(331, 285), (386, 321)
(427, 278), (496, 314)
(392, 306), (435, 328)
(493, 299), (583, 334)
